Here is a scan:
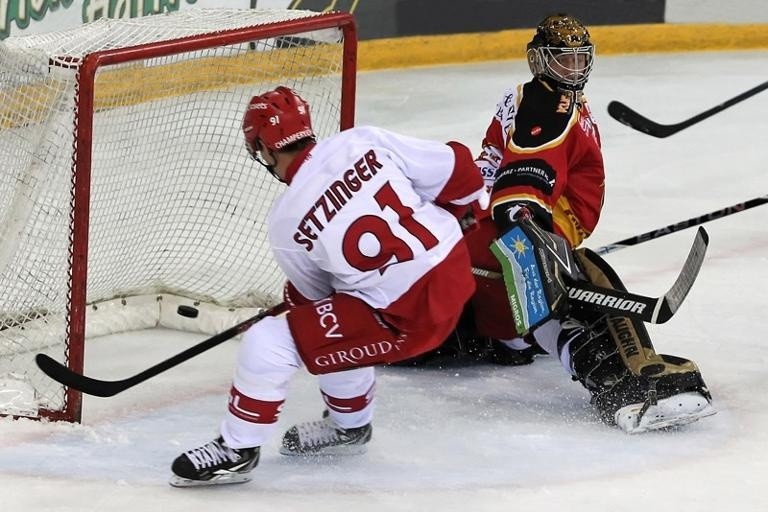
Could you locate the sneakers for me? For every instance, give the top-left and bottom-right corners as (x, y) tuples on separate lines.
(282, 411), (374, 453)
(169, 433), (262, 481)
(592, 355), (709, 430)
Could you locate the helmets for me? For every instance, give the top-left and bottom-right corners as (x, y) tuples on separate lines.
(240, 85), (316, 156)
(525, 15), (596, 78)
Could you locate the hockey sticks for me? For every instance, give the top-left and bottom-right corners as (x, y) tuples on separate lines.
(35, 305), (286, 397)
(468, 225), (706, 322)
(607, 81), (766, 137)
